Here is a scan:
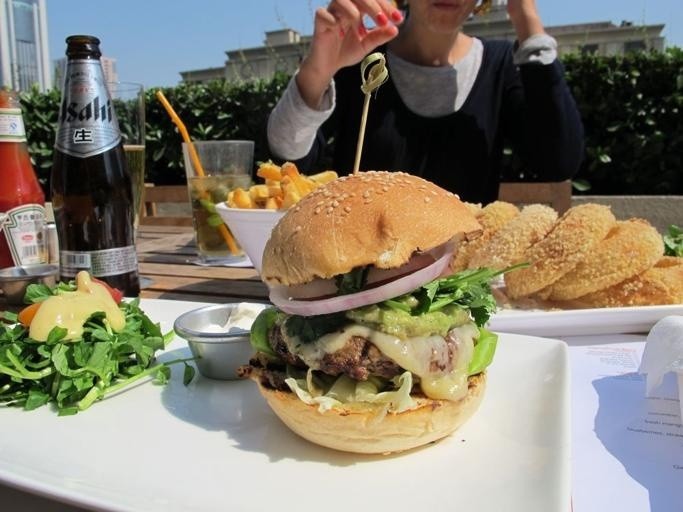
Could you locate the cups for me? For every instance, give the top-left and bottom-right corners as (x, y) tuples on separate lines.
(181, 140), (255, 263)
(105, 82), (145, 252)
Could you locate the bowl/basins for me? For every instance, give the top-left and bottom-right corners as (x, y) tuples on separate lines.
(214, 201), (290, 284)
(1, 264), (60, 308)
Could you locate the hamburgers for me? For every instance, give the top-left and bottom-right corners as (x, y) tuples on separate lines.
(237, 171), (531, 454)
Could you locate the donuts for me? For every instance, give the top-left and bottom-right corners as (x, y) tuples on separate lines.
(464, 201), (682, 309)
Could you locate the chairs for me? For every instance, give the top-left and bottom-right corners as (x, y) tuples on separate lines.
(141, 183), (194, 224)
(501, 178), (572, 218)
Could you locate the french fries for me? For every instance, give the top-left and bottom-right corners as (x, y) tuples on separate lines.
(226, 159), (338, 209)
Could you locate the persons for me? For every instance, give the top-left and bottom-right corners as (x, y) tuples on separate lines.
(266, 1), (585, 204)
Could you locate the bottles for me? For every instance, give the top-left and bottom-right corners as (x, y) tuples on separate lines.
(0, 35), (141, 297)
